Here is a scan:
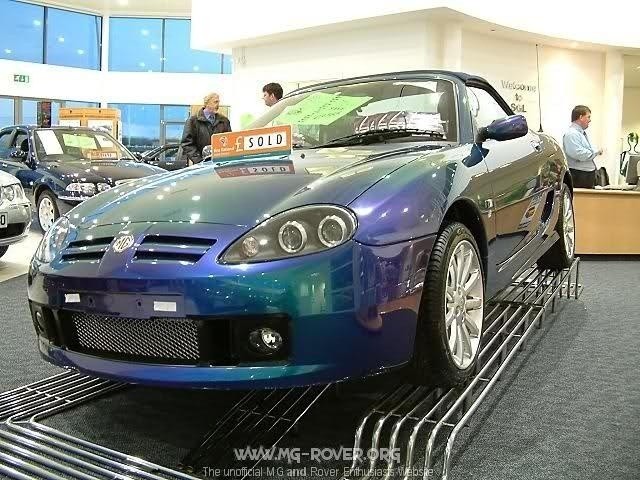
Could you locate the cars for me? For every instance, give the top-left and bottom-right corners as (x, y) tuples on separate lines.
(0, 125), (170, 236)
(0, 169), (34, 258)
(26, 69), (575, 390)
(133, 143), (189, 171)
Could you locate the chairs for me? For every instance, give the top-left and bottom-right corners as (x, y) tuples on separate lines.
(21, 139), (34, 161)
(318, 115), (355, 143)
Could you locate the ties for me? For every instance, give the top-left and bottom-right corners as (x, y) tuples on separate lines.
(210, 116), (214, 124)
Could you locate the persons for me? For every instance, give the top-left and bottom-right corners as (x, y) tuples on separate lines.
(562, 105), (603, 188)
(260, 83), (300, 147)
(180, 92), (232, 164)
